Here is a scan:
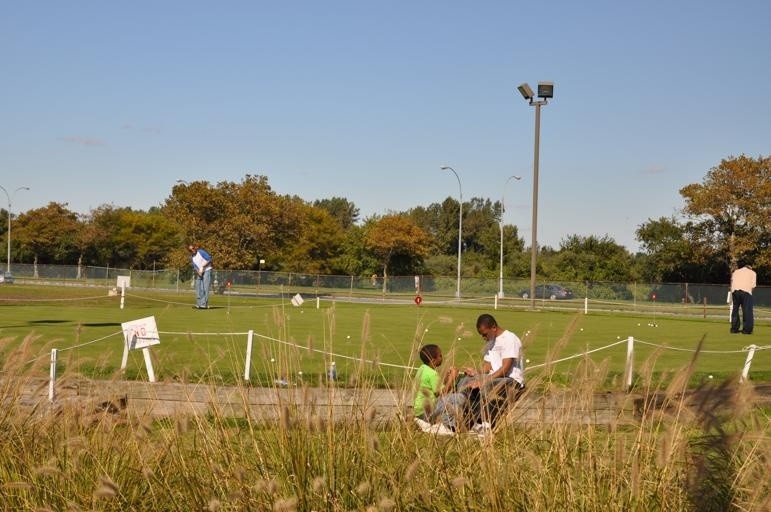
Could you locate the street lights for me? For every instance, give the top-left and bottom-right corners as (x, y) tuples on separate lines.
(517, 80), (554, 311)
(496, 174), (522, 300)
(439, 166), (462, 299)
(0, 184), (31, 272)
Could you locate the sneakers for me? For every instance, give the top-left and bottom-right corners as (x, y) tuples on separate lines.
(414, 417), (454, 435)
(731, 328), (749, 334)
(192, 306), (207, 309)
(469, 421), (490, 437)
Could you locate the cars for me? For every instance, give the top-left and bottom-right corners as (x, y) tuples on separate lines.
(518, 283), (573, 300)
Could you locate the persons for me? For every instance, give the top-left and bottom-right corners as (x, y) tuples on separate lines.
(409, 344), (466, 436)
(188, 244), (213, 309)
(729, 264), (757, 334)
(457, 314), (524, 434)
